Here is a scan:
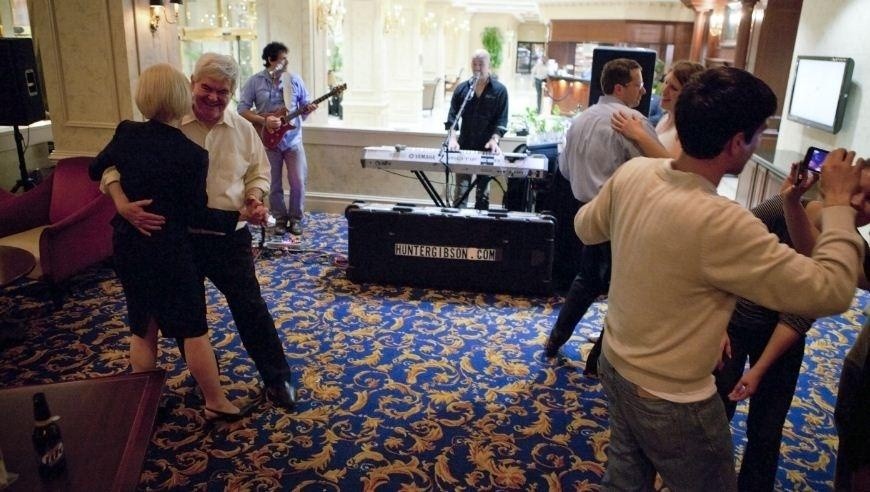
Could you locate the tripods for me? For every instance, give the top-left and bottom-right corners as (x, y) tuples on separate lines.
(9, 127), (40, 195)
(251, 111), (327, 266)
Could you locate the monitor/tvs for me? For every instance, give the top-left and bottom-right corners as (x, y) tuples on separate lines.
(785, 54), (855, 135)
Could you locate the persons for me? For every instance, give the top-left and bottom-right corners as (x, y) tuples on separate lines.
(98, 50), (298, 407)
(611, 61), (707, 161)
(444, 49), (510, 211)
(235, 41), (318, 235)
(542, 58), (658, 381)
(833, 321), (868, 491)
(87, 62), (267, 423)
(708, 157), (870, 491)
(574, 66), (866, 491)
(530, 52), (555, 114)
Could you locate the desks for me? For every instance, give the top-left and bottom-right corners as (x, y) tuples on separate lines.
(0, 371), (167, 492)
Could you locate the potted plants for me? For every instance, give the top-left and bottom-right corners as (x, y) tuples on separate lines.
(327, 46), (342, 91)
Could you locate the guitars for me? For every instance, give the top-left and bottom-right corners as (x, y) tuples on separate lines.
(253, 83), (346, 147)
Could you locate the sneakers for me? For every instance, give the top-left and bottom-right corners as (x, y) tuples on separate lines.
(290, 218), (303, 235)
(276, 218), (287, 235)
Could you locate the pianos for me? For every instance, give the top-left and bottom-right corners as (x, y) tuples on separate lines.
(361, 144), (549, 180)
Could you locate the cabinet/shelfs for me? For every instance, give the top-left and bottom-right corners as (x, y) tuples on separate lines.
(734, 150), (805, 213)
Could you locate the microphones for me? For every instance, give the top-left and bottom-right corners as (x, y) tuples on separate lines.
(467, 70), (481, 101)
(269, 60), (285, 77)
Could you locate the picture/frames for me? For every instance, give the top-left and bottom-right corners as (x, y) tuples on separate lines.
(516, 41), (545, 74)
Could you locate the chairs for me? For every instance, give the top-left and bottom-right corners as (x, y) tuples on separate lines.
(0, 156), (118, 310)
(444, 69), (462, 98)
(422, 77), (441, 113)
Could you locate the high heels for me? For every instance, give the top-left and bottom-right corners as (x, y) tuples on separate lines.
(204, 403), (259, 434)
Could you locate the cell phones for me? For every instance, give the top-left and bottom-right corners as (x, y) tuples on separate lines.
(803, 145), (829, 175)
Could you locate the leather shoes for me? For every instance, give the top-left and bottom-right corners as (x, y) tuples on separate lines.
(588, 331), (601, 343)
(268, 380), (295, 409)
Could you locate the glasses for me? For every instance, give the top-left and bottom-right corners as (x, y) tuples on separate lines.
(617, 82), (643, 90)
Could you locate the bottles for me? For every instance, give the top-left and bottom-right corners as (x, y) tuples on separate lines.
(32, 393), (67, 485)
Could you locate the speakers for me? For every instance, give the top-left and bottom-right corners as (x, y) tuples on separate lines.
(0, 36), (48, 125)
(589, 47), (656, 117)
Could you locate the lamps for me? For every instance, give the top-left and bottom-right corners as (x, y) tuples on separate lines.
(150, 0), (184, 34)
